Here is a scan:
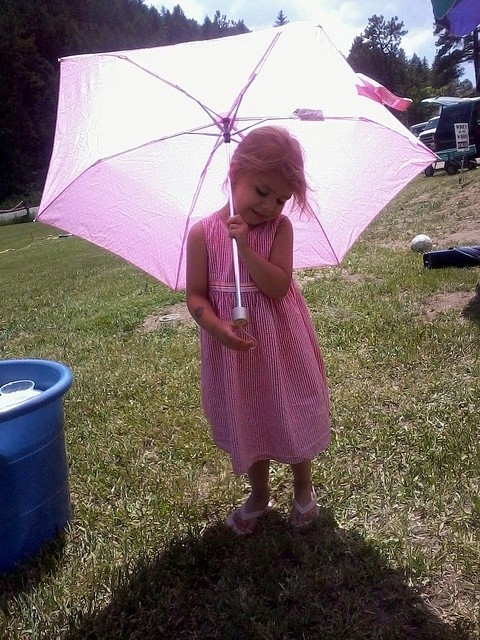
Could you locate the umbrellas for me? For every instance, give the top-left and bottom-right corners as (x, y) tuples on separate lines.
(32, 18), (444, 350)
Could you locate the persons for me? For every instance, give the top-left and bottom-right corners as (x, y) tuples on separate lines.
(185, 126), (332, 535)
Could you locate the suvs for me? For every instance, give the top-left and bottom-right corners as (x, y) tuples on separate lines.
(419, 117), (441, 151)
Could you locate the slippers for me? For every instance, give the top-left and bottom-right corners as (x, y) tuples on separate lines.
(226, 504), (265, 537)
(290, 485), (319, 528)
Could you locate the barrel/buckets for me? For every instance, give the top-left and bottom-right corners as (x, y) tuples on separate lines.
(0, 359), (71, 571)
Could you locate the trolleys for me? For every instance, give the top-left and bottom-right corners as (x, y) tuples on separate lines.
(424, 144), (477, 176)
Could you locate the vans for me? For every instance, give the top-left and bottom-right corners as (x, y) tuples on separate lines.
(419, 96), (480, 160)
